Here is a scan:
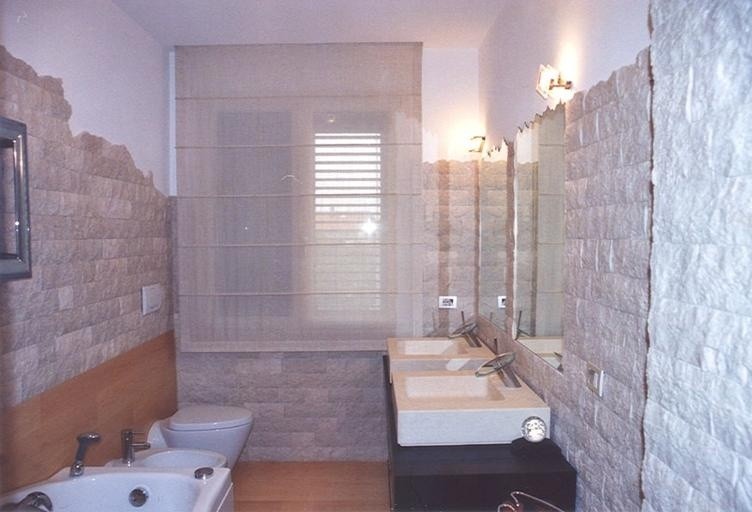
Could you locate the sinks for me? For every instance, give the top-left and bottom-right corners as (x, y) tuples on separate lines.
(103, 448), (228, 470)
(390, 370), (550, 445)
(517, 334), (563, 374)
(384, 335), (497, 383)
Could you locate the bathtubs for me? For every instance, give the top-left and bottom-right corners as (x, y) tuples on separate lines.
(0, 464), (235, 511)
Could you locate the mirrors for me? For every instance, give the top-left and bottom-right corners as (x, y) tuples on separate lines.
(477, 100), (566, 381)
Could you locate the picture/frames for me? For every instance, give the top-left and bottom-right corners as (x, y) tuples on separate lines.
(0, 115), (31, 281)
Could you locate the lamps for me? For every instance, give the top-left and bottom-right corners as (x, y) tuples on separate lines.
(535, 63), (573, 100)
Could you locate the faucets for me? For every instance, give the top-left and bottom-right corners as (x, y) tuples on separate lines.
(122, 428), (150, 465)
(448, 324), (480, 348)
(69, 431), (100, 476)
(551, 351), (563, 374)
(473, 350), (522, 388)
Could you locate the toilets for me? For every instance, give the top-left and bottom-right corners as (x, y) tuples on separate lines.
(147, 403), (254, 473)
